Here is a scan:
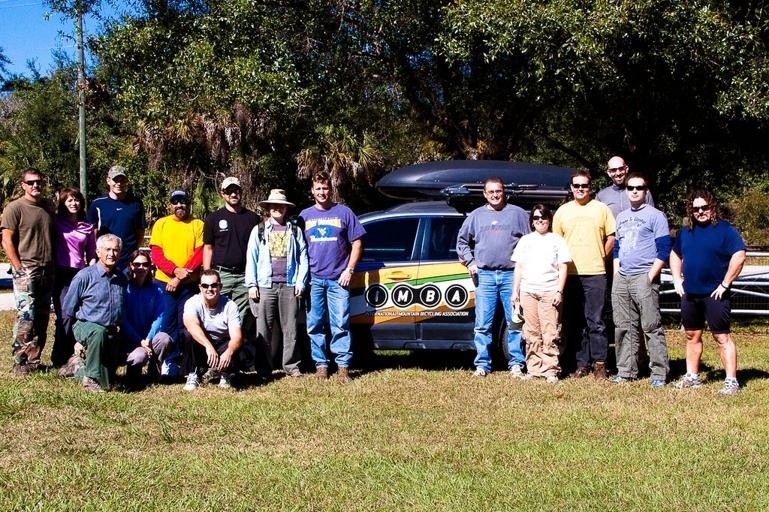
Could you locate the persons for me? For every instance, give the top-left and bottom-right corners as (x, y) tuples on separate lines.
(597, 156), (655, 218)
(550, 168), (617, 380)
(62, 233), (127, 390)
(668, 190), (749, 392)
(203, 175), (261, 329)
(150, 188), (203, 331)
(456, 177), (531, 377)
(246, 189), (308, 380)
(0, 167), (58, 373)
(88, 164), (147, 265)
(297, 172), (366, 379)
(51, 188), (98, 367)
(511, 204), (572, 383)
(119, 250), (179, 384)
(183, 271), (241, 391)
(610, 172), (673, 388)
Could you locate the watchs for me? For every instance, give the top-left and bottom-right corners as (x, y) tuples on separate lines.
(345, 267), (353, 274)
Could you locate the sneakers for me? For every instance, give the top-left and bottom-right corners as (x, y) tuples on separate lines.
(79, 359), (179, 392)
(473, 365), (738, 395)
(216, 374), (236, 392)
(183, 365), (199, 392)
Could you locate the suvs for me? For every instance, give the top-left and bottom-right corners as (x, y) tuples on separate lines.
(349, 157), (581, 358)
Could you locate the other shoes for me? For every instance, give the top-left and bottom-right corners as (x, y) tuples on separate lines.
(59, 364), (75, 376)
(13, 364), (29, 376)
(253, 371), (273, 382)
(32, 363), (46, 372)
(337, 366), (349, 382)
(315, 366), (328, 378)
(288, 370), (303, 377)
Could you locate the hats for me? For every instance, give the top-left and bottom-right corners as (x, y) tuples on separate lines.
(170, 191), (188, 201)
(259, 189), (296, 206)
(222, 177), (242, 189)
(109, 165), (128, 179)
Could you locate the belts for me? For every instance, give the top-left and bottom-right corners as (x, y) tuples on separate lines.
(213, 265), (245, 273)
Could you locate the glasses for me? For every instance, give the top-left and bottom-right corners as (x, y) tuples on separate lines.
(132, 263), (150, 267)
(223, 189), (240, 195)
(201, 283), (219, 288)
(609, 166), (624, 172)
(172, 197), (186, 204)
(692, 205), (709, 212)
(572, 184), (589, 188)
(533, 216), (548, 220)
(626, 186), (645, 191)
(26, 180), (43, 184)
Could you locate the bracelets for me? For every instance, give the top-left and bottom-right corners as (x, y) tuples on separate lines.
(556, 289), (564, 297)
(719, 282), (731, 292)
